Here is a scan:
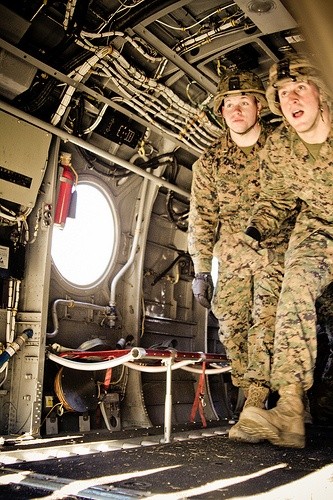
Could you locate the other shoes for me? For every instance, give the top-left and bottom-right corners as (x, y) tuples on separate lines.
(238, 402), (306, 449)
(227, 400), (269, 443)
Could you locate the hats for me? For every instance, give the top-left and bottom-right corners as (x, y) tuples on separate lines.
(213, 70), (267, 112)
(266, 52), (333, 115)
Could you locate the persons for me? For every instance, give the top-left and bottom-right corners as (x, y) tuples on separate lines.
(186, 70), (312, 442)
(238, 58), (333, 447)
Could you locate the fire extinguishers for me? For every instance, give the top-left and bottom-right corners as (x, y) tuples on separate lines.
(54, 151), (78, 230)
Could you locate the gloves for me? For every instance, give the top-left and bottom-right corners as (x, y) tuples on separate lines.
(192, 273), (215, 309)
